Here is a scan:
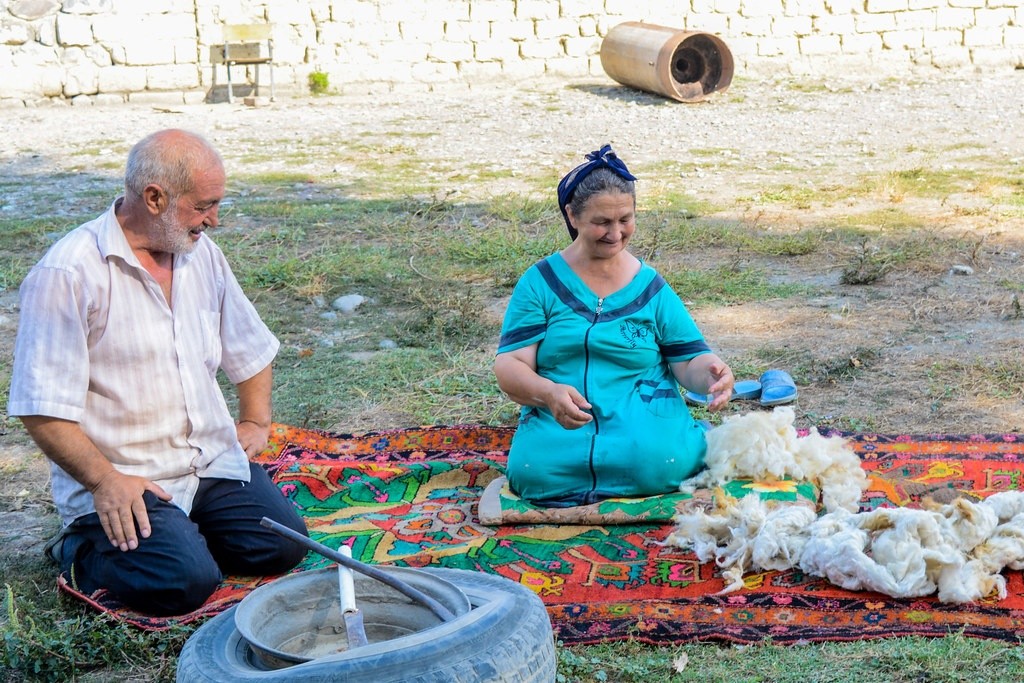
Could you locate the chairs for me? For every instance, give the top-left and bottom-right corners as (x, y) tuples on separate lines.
(221, 22), (274, 105)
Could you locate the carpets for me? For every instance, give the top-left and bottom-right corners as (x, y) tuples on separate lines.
(52, 419), (1024, 647)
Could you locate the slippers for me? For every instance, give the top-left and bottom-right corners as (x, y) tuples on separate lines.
(684, 379), (761, 406)
(758, 370), (798, 406)
(43, 526), (74, 560)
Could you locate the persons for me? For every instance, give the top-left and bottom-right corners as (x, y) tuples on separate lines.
(493, 144), (735, 509)
(6, 130), (309, 619)
